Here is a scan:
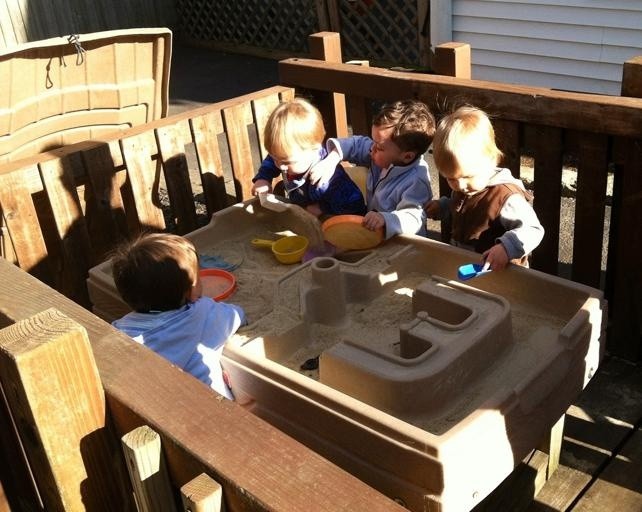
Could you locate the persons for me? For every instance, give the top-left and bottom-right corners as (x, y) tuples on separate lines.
(251, 95), (367, 217)
(308, 96), (439, 244)
(422, 101), (547, 272)
(109, 233), (250, 403)
